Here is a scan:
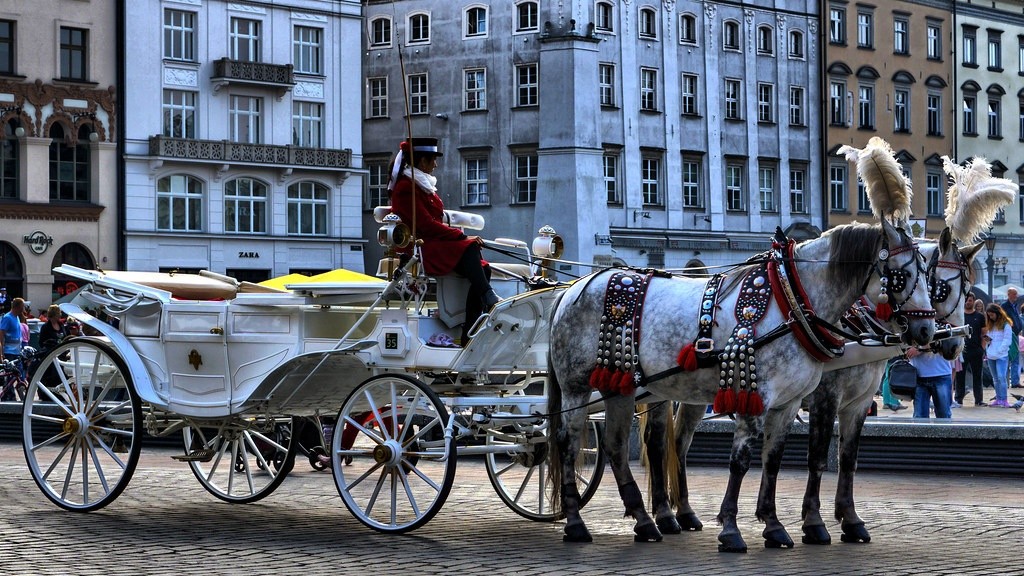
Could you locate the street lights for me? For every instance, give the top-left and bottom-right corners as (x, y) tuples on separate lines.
(982, 227), (997, 302)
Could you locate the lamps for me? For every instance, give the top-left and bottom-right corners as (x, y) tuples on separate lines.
(544, 19), (596, 38)
(640, 250), (646, 255)
(0, 105), (25, 137)
(74, 112), (98, 142)
(694, 214), (711, 226)
(435, 112), (448, 119)
(694, 251), (700, 256)
(634, 210), (651, 222)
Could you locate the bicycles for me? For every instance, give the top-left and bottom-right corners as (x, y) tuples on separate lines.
(0, 346), (47, 401)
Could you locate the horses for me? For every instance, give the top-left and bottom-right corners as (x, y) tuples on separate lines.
(637, 225), (987, 547)
(541, 209), (937, 552)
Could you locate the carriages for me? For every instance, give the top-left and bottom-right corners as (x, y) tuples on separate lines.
(20, 136), (1019, 554)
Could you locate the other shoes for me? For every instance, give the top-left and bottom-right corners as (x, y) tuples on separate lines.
(975, 401), (987, 407)
(929, 400), (935, 408)
(952, 401), (963, 408)
(1012, 383), (1024, 388)
(882, 401), (908, 409)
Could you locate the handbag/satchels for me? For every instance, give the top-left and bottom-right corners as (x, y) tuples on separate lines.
(888, 357), (917, 395)
(1003, 321), (1018, 361)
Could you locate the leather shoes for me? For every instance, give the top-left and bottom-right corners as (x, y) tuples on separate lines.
(460, 322), (478, 348)
(481, 289), (504, 308)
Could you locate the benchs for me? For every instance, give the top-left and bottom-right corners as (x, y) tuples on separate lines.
(374, 204), (534, 284)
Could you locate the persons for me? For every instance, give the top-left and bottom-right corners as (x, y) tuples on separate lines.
(954, 292), (988, 407)
(974, 298), (984, 312)
(985, 303), (1013, 407)
(882, 355), (908, 409)
(907, 344), (952, 418)
(0, 298), (120, 402)
(387, 138), (504, 347)
(1000, 287), (1024, 388)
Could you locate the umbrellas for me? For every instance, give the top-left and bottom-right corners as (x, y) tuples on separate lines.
(974, 282), (1024, 301)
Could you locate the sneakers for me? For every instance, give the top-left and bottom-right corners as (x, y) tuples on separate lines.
(1001, 400), (1009, 407)
(988, 399), (1002, 406)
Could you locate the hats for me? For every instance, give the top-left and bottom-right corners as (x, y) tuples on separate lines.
(0, 288), (6, 290)
(400, 137), (443, 156)
